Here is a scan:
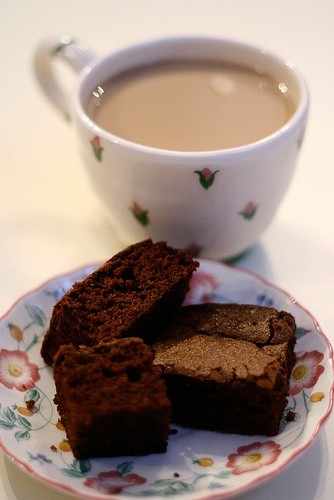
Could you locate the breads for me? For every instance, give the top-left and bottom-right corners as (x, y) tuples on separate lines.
(150, 302), (298, 438)
(53, 337), (169, 461)
(40, 238), (201, 367)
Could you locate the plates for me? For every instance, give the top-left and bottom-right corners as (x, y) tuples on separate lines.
(0, 256), (334, 500)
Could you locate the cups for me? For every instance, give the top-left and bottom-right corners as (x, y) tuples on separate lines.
(32, 30), (309, 260)
